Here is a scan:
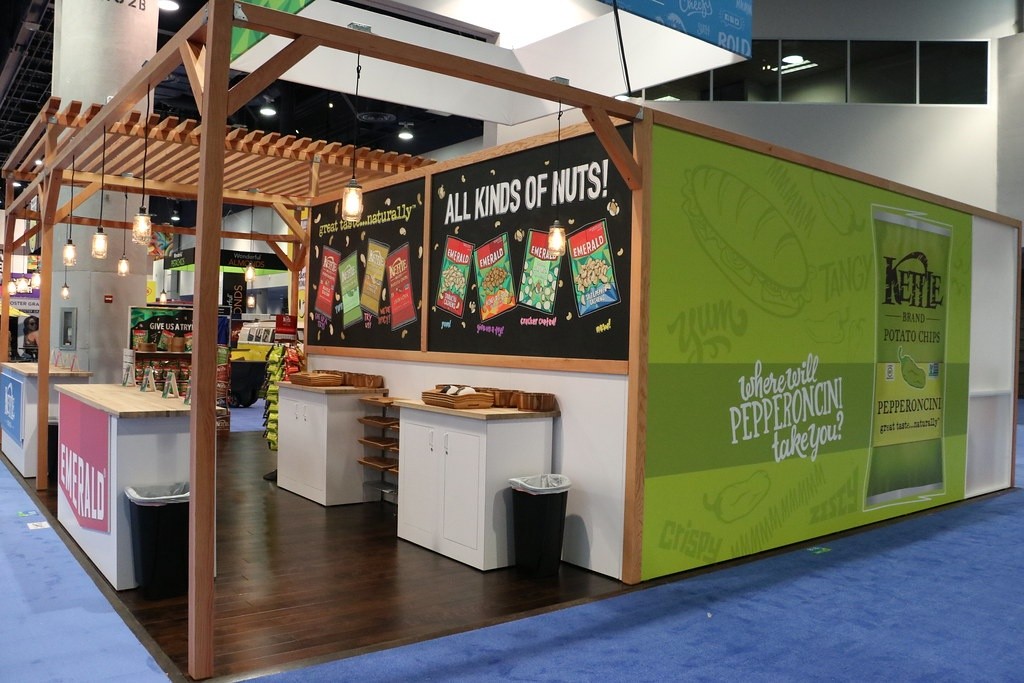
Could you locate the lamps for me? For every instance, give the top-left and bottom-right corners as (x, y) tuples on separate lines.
(7, 210), (17, 295)
(160, 232), (167, 304)
(16, 201), (32, 294)
(547, 97), (571, 257)
(91, 124), (108, 260)
(246, 201), (259, 284)
(343, 132), (363, 223)
(117, 187), (132, 278)
(61, 223), (71, 300)
(63, 155), (80, 268)
(397, 120), (416, 142)
(248, 282), (256, 309)
(259, 94), (279, 118)
(131, 84), (157, 249)
(31, 194), (41, 289)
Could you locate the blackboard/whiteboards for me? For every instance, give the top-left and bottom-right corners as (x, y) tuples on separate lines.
(303, 117), (634, 374)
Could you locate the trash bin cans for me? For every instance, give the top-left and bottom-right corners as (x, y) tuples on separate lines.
(48, 416), (59, 488)
(124, 481), (190, 602)
(509, 473), (571, 579)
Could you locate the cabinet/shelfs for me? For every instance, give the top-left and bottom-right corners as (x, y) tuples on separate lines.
(276, 381), (389, 506)
(357, 394), (404, 496)
(392, 400), (561, 571)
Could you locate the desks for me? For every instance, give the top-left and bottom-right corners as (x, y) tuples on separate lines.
(53, 383), (227, 591)
(0, 363), (94, 478)
